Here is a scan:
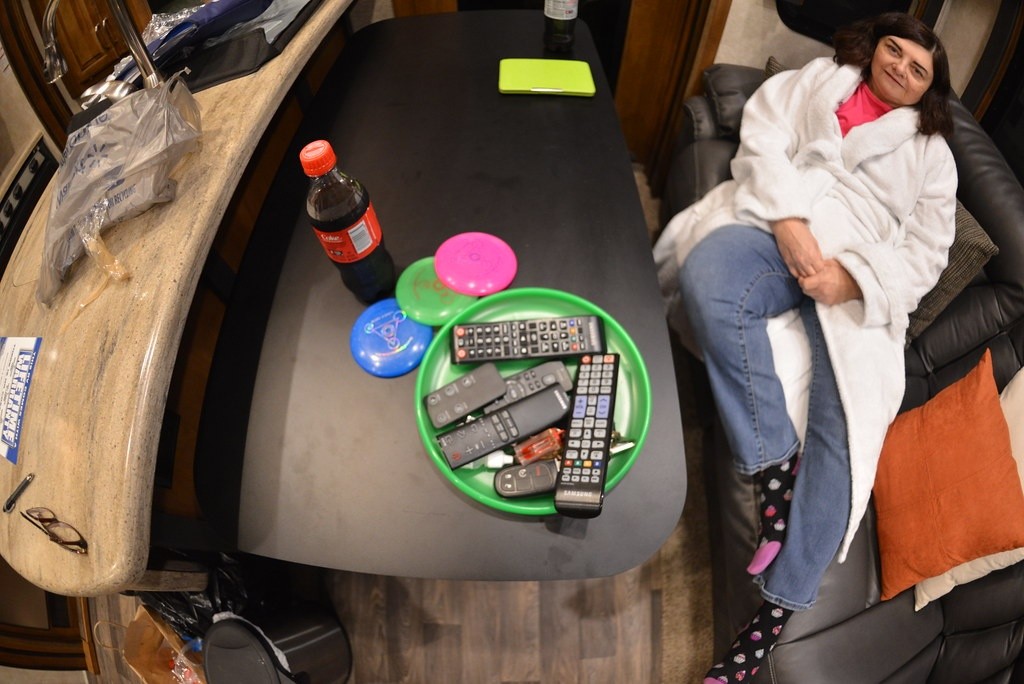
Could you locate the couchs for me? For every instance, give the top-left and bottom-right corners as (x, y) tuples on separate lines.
(670, 57), (1024, 684)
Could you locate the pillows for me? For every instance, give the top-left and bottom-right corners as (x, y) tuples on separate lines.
(914, 366), (1024, 611)
(905, 197), (999, 347)
(873, 348), (1024, 600)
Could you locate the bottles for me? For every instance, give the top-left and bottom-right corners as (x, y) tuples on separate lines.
(299, 138), (394, 295)
(542, 0), (579, 51)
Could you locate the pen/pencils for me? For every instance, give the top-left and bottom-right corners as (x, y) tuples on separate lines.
(1, 472), (35, 514)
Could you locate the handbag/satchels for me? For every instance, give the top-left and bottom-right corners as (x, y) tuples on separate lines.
(93, 604), (208, 684)
(33, 72), (203, 313)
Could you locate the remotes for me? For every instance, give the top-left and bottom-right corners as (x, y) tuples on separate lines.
(421, 313), (622, 521)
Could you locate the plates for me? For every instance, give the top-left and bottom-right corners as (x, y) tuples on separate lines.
(413, 286), (651, 515)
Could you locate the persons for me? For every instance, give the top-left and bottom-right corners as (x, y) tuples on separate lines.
(651, 15), (960, 684)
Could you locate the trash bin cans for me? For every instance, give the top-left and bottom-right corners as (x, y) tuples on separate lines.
(191, 600), (350, 683)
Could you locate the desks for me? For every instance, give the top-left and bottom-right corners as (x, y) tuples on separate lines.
(239, 9), (687, 579)
